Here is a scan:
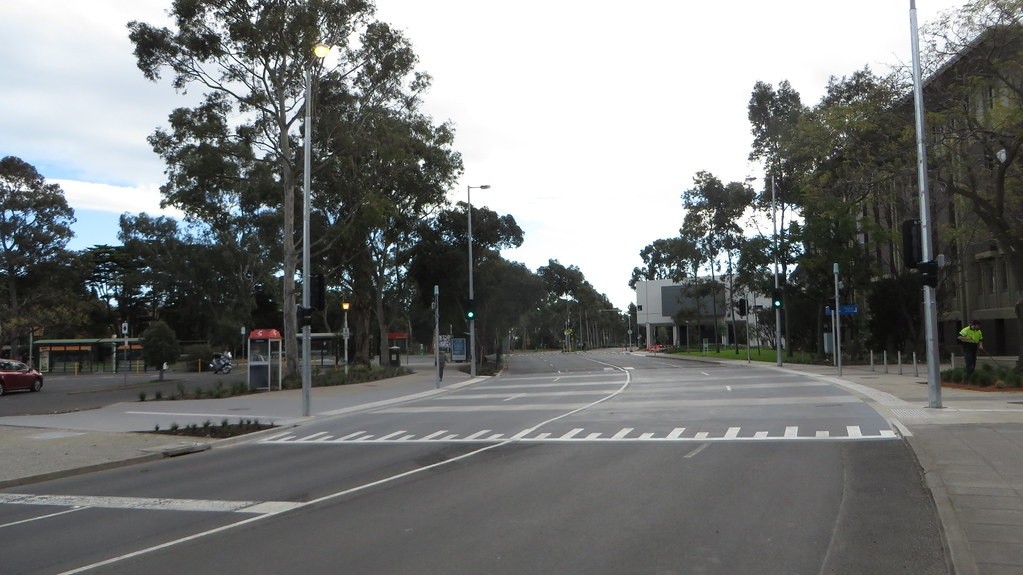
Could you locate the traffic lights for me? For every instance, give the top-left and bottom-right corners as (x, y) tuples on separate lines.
(828, 297), (836, 310)
(310, 273), (325, 311)
(916, 261), (938, 289)
(736, 299), (746, 317)
(296, 305), (315, 329)
(772, 288), (783, 309)
(466, 299), (477, 320)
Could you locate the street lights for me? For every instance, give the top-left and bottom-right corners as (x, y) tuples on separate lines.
(744, 175), (782, 367)
(342, 299), (352, 376)
(467, 184), (491, 378)
(301, 43), (330, 417)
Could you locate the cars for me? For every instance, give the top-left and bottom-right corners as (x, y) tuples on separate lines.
(0, 358), (43, 396)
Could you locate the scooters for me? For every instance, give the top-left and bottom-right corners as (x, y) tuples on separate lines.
(211, 348), (233, 375)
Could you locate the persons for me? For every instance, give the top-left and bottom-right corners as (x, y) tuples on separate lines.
(435, 349), (450, 382)
(955, 320), (984, 381)
(224, 348), (231, 358)
